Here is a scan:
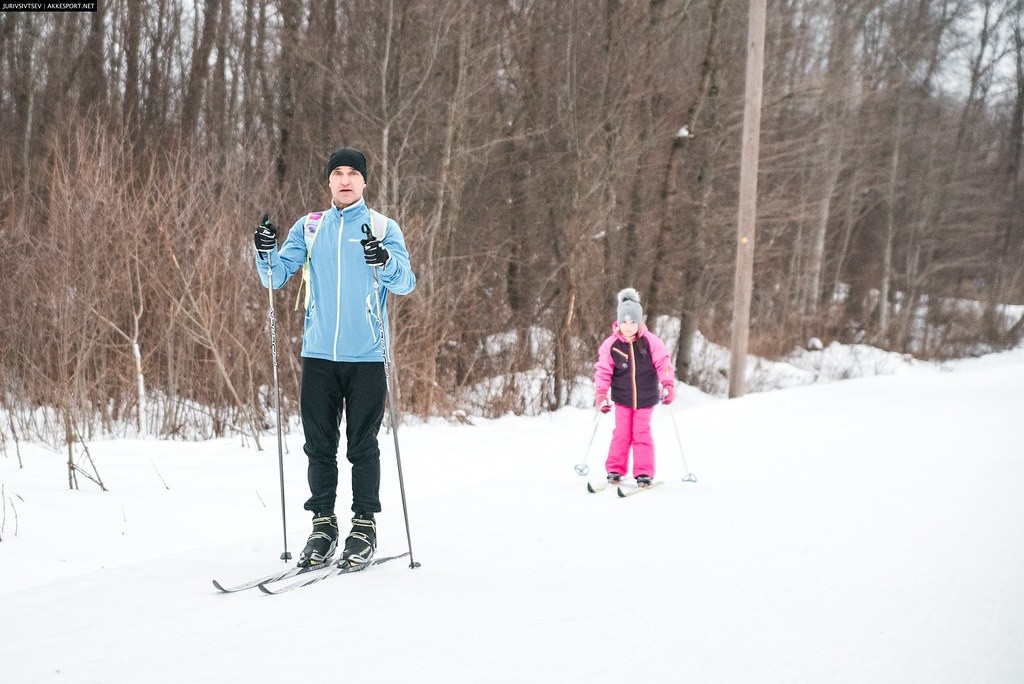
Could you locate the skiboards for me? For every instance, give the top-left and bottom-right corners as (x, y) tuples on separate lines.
(587, 472), (667, 499)
(210, 549), (413, 596)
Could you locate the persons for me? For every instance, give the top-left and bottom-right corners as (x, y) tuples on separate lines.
(594, 288), (675, 488)
(253, 149), (416, 568)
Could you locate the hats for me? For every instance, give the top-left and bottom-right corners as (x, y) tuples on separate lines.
(616, 288), (643, 331)
(327, 149), (368, 183)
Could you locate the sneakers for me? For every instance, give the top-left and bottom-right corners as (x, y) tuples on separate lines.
(297, 513), (338, 567)
(637, 476), (651, 488)
(607, 473), (620, 485)
(337, 512), (377, 569)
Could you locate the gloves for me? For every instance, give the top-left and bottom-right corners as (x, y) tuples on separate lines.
(660, 384), (674, 405)
(254, 223), (277, 253)
(596, 393), (612, 413)
(360, 237), (391, 268)
